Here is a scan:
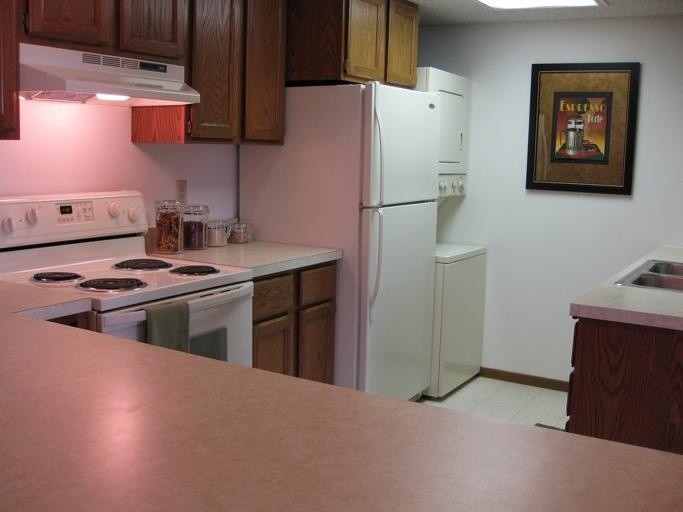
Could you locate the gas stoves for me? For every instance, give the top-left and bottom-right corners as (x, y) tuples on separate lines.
(0, 252), (253, 311)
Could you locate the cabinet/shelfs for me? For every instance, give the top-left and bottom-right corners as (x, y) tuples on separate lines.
(252, 261), (336, 384)
(131, 0), (285, 145)
(285, 0), (420, 89)
(26, 0), (186, 63)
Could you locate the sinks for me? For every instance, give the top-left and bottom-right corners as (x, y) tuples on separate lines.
(613, 259), (683, 293)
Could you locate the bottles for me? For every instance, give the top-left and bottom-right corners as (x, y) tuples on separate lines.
(151, 198), (254, 254)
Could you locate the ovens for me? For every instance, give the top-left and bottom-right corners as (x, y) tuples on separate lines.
(80, 281), (254, 370)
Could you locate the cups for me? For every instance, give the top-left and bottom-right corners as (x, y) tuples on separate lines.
(559, 127), (584, 156)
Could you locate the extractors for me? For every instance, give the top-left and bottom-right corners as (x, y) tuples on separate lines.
(17, 42), (202, 111)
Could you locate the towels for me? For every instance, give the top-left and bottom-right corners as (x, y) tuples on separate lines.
(142, 300), (190, 354)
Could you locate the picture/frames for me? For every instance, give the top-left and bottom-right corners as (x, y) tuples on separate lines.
(526, 61), (640, 195)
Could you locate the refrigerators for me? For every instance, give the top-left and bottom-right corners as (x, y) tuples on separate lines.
(235, 80), (438, 403)
